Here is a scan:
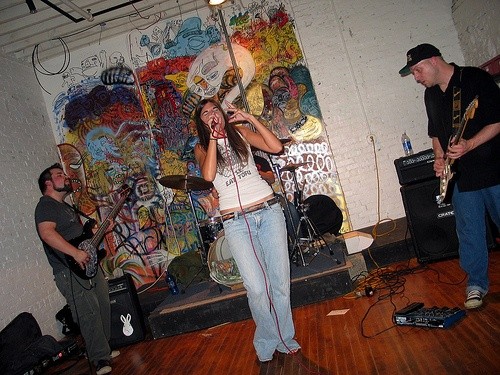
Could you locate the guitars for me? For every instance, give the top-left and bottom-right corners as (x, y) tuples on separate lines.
(65, 186), (132, 280)
(439, 98), (479, 204)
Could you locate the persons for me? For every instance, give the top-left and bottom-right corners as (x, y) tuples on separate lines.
(398, 44), (500, 309)
(34, 163), (121, 375)
(195, 99), (301, 361)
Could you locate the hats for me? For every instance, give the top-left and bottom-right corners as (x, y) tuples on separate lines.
(398, 43), (442, 74)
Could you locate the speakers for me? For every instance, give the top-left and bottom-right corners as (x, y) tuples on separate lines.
(399, 176), (496, 266)
(108, 276), (147, 349)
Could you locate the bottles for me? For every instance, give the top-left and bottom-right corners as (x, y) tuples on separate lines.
(401, 133), (413, 155)
(166, 271), (179, 295)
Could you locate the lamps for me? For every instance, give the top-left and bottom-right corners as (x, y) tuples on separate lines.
(208, 0), (226, 5)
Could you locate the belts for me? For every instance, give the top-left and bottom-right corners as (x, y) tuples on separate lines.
(221, 196), (280, 222)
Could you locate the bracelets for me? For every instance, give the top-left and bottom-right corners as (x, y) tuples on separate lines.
(209, 138), (217, 140)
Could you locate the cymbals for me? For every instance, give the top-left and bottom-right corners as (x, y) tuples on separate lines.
(273, 161), (311, 174)
(278, 139), (291, 144)
(159, 175), (214, 191)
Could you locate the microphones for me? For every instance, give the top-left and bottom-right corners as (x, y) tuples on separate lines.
(211, 121), (215, 128)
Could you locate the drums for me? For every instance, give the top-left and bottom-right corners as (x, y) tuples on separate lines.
(207, 229), (244, 285)
(196, 217), (223, 260)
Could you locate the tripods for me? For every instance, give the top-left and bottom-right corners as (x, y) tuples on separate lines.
(266, 154), (340, 267)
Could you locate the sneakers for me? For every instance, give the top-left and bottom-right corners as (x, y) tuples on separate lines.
(110, 350), (120, 358)
(464, 290), (483, 309)
(96, 359), (111, 374)
